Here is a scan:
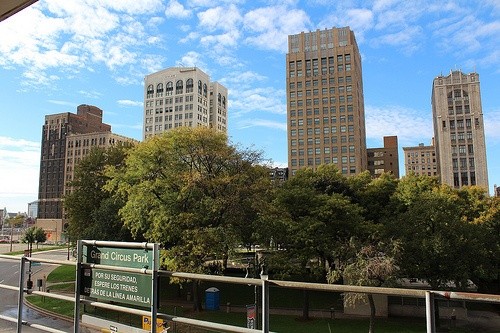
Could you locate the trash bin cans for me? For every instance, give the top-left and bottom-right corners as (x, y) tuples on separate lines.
(205, 287), (220, 310)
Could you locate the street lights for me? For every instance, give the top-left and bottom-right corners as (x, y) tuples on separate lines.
(63, 226), (72, 262)
(24, 233), (38, 295)
(245, 254), (269, 330)
(52, 220), (58, 243)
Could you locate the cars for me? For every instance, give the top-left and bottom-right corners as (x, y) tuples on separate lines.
(0, 236), (38, 244)
